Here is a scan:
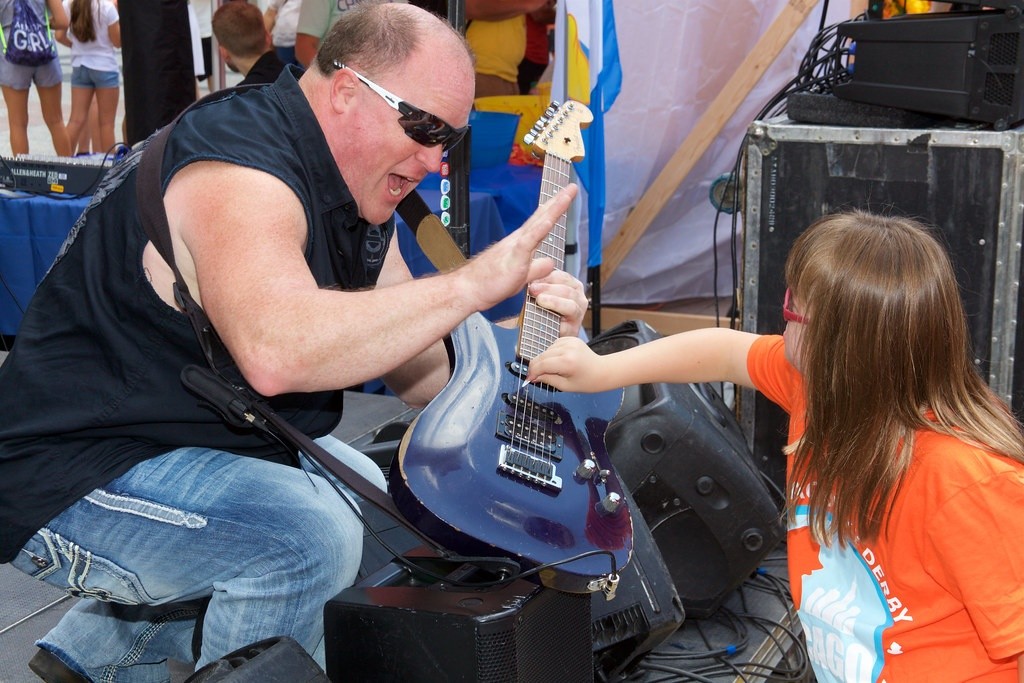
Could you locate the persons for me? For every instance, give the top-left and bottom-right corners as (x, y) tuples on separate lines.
(526, 215), (1024, 683)
(1, 0), (587, 681)
(75, 1), (121, 155)
(211, 2), (287, 88)
(462, 1), (551, 97)
(293, 2), (411, 67)
(0, 0), (72, 158)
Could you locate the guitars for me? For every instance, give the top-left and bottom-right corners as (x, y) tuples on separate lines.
(389, 98), (633, 605)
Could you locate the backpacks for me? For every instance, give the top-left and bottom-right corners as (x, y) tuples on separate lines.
(1, 0), (58, 64)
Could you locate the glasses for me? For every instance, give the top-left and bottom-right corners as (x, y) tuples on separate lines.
(334, 61), (469, 152)
(783, 289), (809, 324)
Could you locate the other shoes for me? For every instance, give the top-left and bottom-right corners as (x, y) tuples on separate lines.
(28, 648), (87, 683)
